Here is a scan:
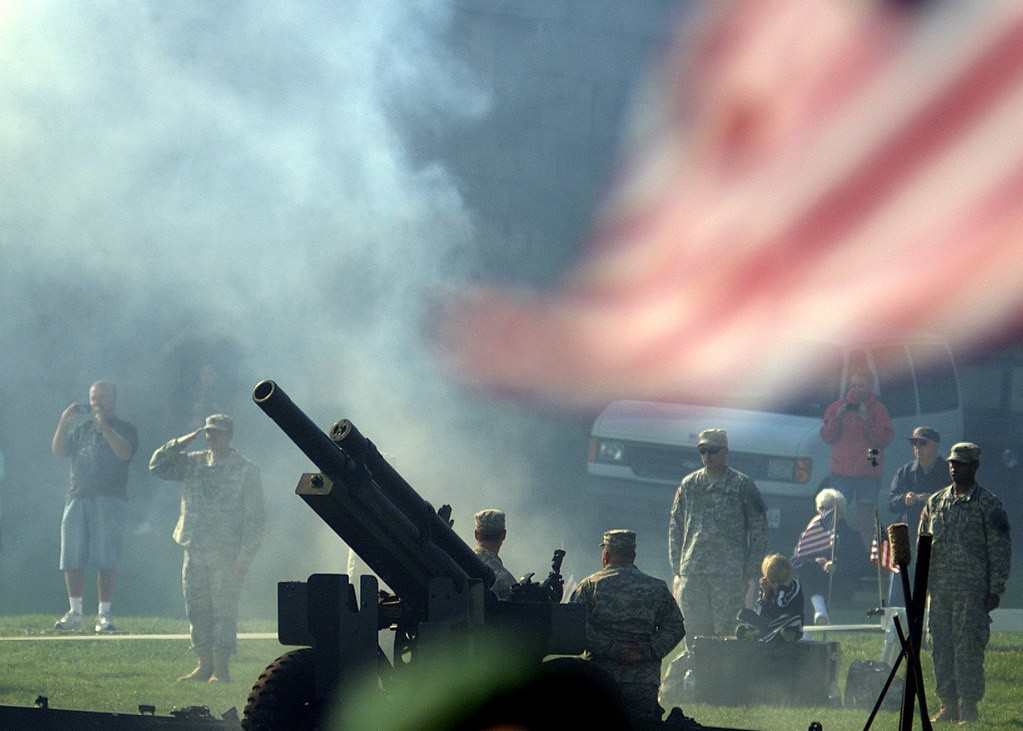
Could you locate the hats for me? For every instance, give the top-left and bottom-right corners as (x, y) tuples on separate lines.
(203, 414), (234, 433)
(907, 427), (941, 444)
(598, 529), (637, 550)
(474, 509), (505, 535)
(699, 429), (728, 449)
(945, 442), (981, 464)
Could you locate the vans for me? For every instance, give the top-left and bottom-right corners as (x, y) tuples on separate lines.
(583, 322), (962, 556)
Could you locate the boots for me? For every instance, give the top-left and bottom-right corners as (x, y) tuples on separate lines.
(176, 648), (214, 682)
(959, 696), (978, 723)
(930, 695), (960, 723)
(208, 650), (230, 683)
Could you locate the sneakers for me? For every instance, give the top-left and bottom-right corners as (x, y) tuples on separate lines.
(94, 614), (117, 632)
(780, 627), (803, 642)
(736, 623), (760, 642)
(53, 609), (86, 632)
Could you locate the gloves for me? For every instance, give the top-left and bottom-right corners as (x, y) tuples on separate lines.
(607, 641), (636, 665)
(984, 592), (1000, 614)
(438, 504), (454, 527)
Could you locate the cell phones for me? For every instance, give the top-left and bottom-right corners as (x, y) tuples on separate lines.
(73, 405), (91, 415)
(846, 403), (860, 410)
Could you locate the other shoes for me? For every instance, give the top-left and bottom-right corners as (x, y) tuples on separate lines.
(812, 612), (833, 625)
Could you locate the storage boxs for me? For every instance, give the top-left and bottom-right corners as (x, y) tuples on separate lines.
(693, 635), (843, 706)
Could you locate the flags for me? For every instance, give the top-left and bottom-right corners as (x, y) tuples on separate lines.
(796, 507), (836, 559)
(869, 516), (900, 573)
(421, 0), (1023, 411)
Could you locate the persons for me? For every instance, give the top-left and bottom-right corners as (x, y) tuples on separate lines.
(148, 413), (265, 684)
(886, 426), (953, 650)
(471, 509), (518, 601)
(51, 379), (138, 632)
(913, 443), (1011, 725)
(567, 529), (686, 702)
(668, 429), (768, 650)
(735, 554), (804, 644)
(820, 368), (894, 536)
(345, 425), (407, 610)
(793, 488), (867, 641)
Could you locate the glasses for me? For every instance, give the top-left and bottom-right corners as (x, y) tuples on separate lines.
(909, 439), (929, 447)
(850, 381), (870, 389)
(699, 446), (722, 456)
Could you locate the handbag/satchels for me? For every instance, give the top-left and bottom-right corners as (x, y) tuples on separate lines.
(844, 659), (904, 712)
